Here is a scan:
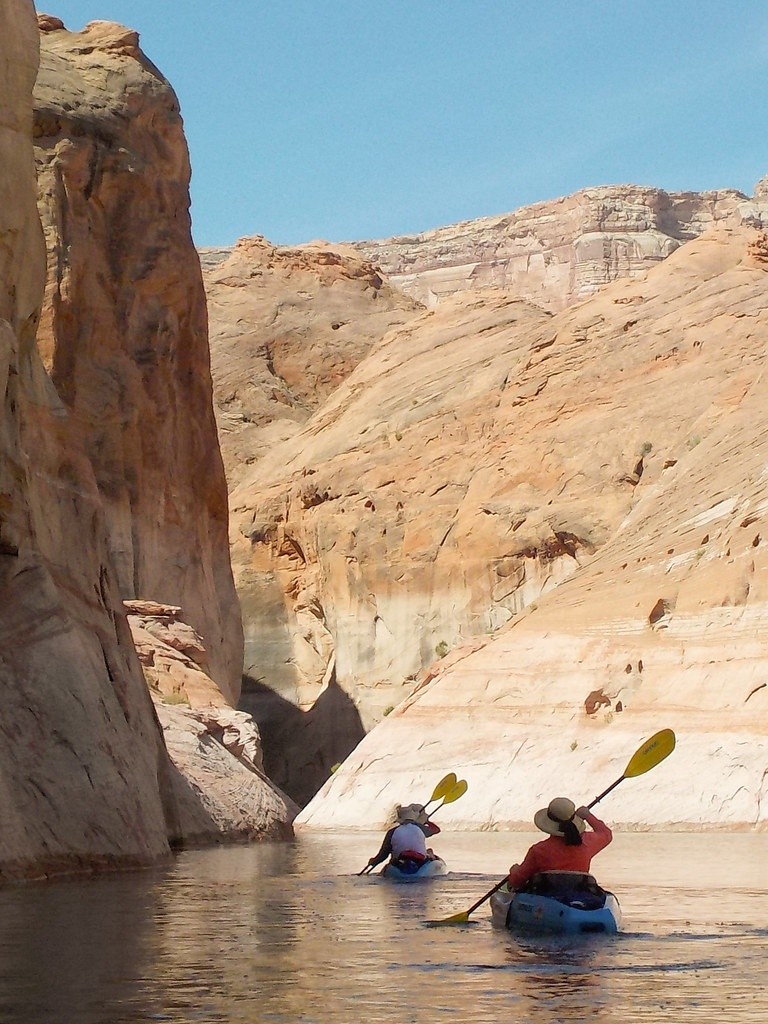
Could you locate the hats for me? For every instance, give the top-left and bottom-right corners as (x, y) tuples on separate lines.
(392, 805), (419, 823)
(534, 796), (586, 846)
(412, 804), (429, 825)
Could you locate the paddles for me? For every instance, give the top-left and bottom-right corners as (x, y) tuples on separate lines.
(353, 770), (473, 877)
(438, 728), (680, 924)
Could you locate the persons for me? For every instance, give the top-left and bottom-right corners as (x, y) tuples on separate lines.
(509, 798), (613, 897)
(368, 803), (442, 870)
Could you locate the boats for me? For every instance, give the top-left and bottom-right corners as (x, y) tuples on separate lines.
(487, 888), (624, 939)
(382, 855), (451, 883)
